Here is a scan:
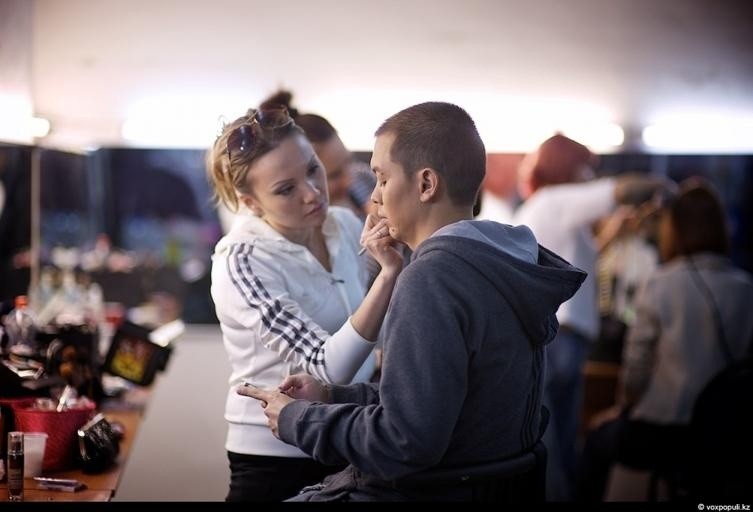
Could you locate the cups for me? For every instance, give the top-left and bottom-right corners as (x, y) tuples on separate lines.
(23, 431), (48, 479)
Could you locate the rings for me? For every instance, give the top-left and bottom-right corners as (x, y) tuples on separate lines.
(375, 231), (383, 237)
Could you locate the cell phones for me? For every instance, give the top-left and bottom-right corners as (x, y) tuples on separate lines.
(244, 382), (268, 405)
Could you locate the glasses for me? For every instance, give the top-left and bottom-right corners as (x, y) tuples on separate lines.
(227, 105), (294, 166)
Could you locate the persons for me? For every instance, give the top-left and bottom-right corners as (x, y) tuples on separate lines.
(504, 129), (753, 511)
(206, 102), (408, 501)
(260, 91), (377, 226)
(234, 99), (590, 503)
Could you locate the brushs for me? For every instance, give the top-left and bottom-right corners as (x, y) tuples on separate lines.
(357, 247), (366, 256)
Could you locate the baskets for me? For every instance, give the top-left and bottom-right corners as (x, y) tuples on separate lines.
(11, 396), (97, 471)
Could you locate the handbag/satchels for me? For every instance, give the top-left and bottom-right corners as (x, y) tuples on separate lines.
(76, 414), (119, 473)
